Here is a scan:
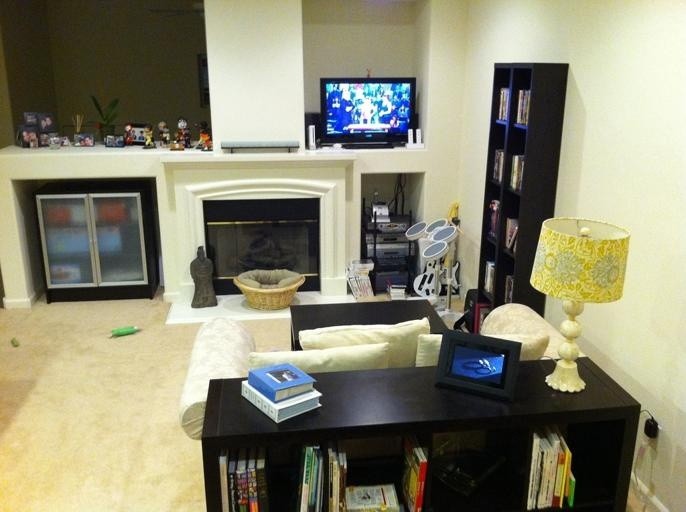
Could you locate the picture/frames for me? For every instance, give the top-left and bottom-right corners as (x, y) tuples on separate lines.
(131, 123), (145, 146)
(434, 328), (523, 405)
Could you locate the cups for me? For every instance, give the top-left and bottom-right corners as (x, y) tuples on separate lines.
(48, 133), (62, 149)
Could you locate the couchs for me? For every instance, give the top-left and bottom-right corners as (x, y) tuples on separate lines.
(180, 303), (588, 440)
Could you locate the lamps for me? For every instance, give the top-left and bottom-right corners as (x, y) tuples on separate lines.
(529, 217), (632, 393)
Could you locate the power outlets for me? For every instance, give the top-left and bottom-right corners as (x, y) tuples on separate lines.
(646, 425), (663, 453)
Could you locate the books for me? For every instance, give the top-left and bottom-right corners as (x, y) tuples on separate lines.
(241, 361), (323, 424)
(527, 422), (576, 511)
(505, 217), (517, 254)
(504, 275), (515, 305)
(385, 278), (407, 300)
(294, 432), (427, 512)
(220, 439), (268, 512)
(484, 261), (495, 295)
(346, 258), (375, 299)
(492, 148), (504, 184)
(516, 90), (530, 127)
(498, 86), (507, 121)
(509, 153), (525, 190)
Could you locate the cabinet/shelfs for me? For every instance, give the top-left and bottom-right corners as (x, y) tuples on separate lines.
(33, 179), (160, 304)
(478, 61), (570, 318)
(200, 356), (642, 512)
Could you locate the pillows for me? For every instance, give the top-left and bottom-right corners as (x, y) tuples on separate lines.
(299, 316), (432, 369)
(247, 342), (391, 373)
(416, 333), (550, 368)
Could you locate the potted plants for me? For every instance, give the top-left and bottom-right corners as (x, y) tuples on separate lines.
(90, 94), (121, 140)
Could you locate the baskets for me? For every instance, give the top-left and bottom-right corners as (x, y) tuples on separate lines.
(234, 271), (305, 310)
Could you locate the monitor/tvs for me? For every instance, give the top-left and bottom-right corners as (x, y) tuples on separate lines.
(319, 77), (415, 143)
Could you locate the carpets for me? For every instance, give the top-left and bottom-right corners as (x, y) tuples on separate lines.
(164, 291), (358, 326)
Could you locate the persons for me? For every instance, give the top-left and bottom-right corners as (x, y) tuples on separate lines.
(121, 115), (212, 151)
